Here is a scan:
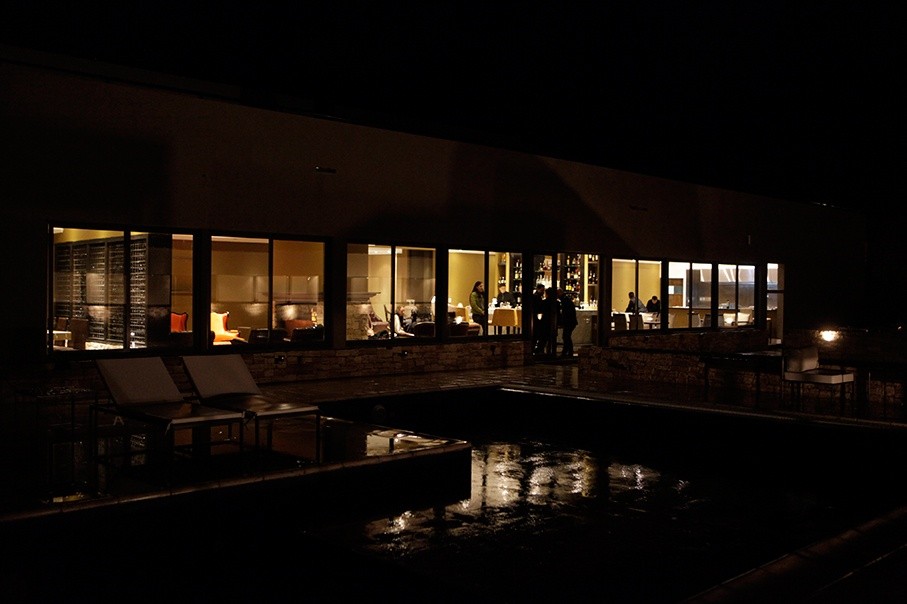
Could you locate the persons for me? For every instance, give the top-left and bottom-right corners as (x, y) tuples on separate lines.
(497, 285), (516, 334)
(556, 289), (578, 358)
(533, 284), (546, 354)
(394, 306), (415, 336)
(647, 296), (660, 313)
(469, 280), (486, 327)
(625, 293), (647, 312)
(537, 288), (560, 358)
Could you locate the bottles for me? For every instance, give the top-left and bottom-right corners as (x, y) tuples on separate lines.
(535, 255), (596, 294)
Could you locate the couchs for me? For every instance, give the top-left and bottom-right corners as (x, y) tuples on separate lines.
(91, 357), (248, 479)
(180, 353), (323, 470)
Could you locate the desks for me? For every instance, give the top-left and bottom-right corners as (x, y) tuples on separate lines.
(0, 377), (101, 499)
